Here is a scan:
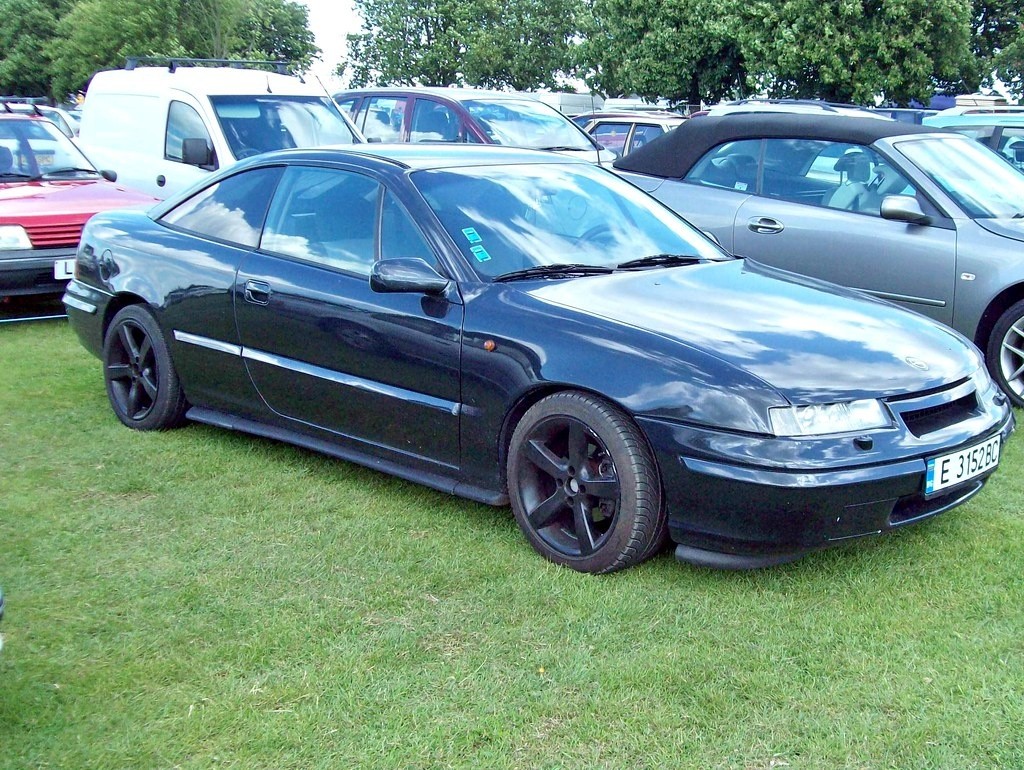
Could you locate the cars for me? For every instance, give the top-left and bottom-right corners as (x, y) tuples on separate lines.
(536, 92), (1024, 218)
(0, 99), (167, 315)
(613, 105), (1024, 414)
(61, 142), (1016, 574)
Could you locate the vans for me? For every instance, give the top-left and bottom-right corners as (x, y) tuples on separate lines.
(79, 57), (372, 202)
(331, 86), (617, 174)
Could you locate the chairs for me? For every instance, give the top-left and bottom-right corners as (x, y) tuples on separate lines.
(312, 194), (399, 275)
(424, 111), (449, 138)
(822, 151), (909, 221)
(0, 145), (26, 176)
(702, 152), (791, 195)
